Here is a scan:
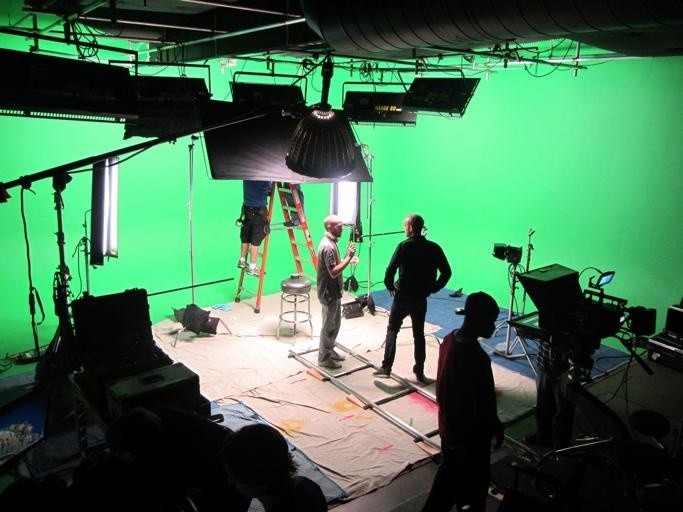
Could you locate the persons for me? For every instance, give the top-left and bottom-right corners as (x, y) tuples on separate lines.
(282, 182), (305, 225)
(210, 423), (328, 512)
(421, 291), (505, 512)
(236, 180), (272, 276)
(315, 214), (361, 369)
(373, 214), (452, 382)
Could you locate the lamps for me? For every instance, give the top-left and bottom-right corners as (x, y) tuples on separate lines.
(286, 55), (359, 179)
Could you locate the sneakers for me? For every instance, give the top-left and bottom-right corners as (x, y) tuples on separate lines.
(331, 352), (344, 359)
(318, 361), (339, 368)
(282, 222), (303, 228)
(238, 261), (252, 271)
(413, 365), (422, 379)
(247, 268), (265, 275)
(373, 367), (391, 378)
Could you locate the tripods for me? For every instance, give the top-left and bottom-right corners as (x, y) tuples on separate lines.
(477, 265), (538, 378)
(493, 228), (535, 356)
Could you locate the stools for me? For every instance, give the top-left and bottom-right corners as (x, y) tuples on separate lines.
(277, 277), (314, 337)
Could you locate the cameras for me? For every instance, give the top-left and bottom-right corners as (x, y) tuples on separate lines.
(492, 244), (523, 267)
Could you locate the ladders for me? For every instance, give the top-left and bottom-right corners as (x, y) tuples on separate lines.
(235, 182), (317, 313)
